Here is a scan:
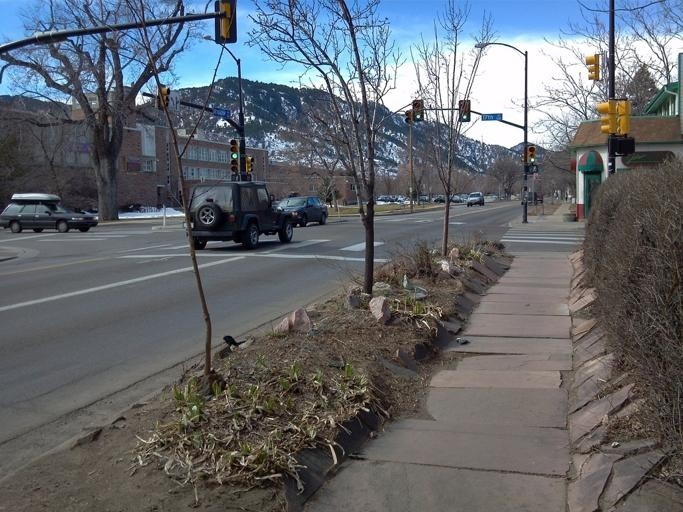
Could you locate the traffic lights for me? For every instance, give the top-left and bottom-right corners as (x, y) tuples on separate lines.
(529, 165), (538, 172)
(412, 100), (424, 123)
(527, 146), (536, 164)
(247, 156), (255, 174)
(613, 102), (631, 136)
(230, 138), (239, 174)
(405, 110), (412, 123)
(597, 100), (614, 134)
(585, 54), (600, 81)
(460, 100), (470, 122)
(157, 85), (170, 109)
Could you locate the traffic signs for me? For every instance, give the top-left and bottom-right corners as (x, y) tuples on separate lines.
(212, 108), (229, 118)
(482, 113), (503, 122)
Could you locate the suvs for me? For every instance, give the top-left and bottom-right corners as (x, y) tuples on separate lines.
(466, 192), (485, 208)
(186, 177), (294, 248)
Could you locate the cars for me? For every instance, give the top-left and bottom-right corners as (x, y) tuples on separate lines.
(277, 196), (330, 226)
(341, 198), (363, 205)
(376, 193), (468, 204)
(0, 193), (99, 233)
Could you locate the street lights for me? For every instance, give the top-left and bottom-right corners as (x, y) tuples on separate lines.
(473, 42), (529, 224)
(202, 35), (247, 182)
